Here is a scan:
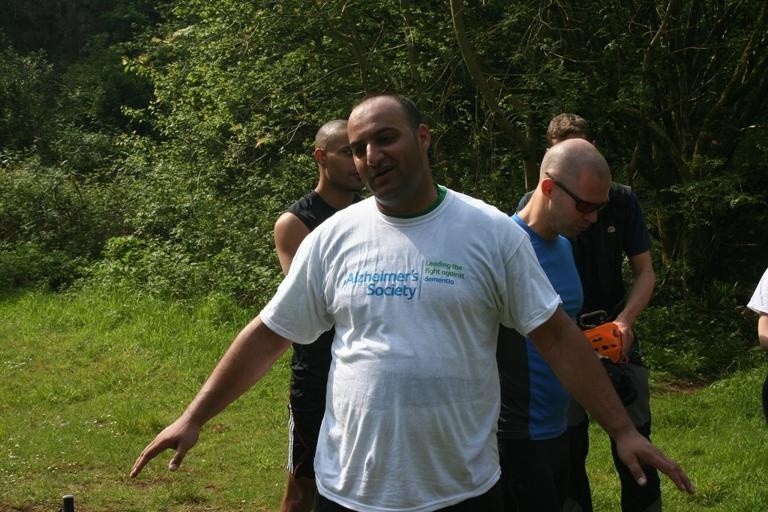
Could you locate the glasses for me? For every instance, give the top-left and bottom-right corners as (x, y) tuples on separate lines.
(545, 172), (609, 214)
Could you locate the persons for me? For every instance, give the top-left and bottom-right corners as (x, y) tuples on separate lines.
(483, 137), (615, 511)
(542, 111), (664, 512)
(129, 89), (699, 511)
(743, 264), (768, 430)
(271, 116), (374, 511)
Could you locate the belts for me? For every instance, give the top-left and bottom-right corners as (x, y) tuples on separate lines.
(578, 297), (628, 329)
